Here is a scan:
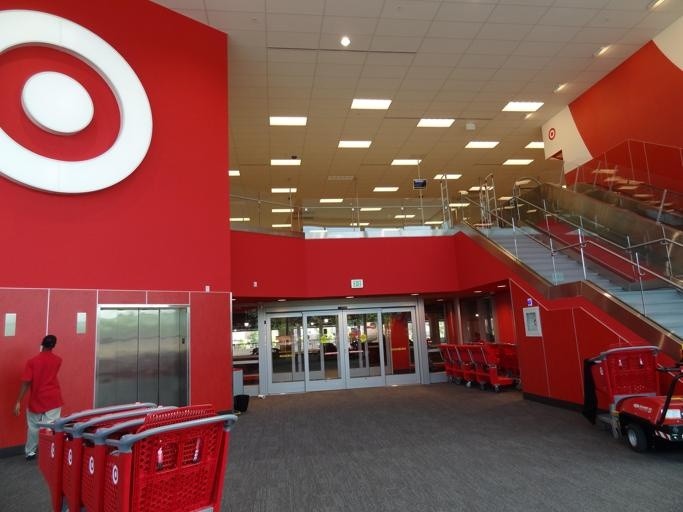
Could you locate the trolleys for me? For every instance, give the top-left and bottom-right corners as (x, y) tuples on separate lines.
(584, 346), (683, 454)
(37, 403), (239, 512)
(436, 343), (521, 391)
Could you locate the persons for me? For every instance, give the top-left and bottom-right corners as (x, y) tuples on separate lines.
(13, 335), (64, 460)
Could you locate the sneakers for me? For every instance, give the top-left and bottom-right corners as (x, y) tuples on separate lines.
(25, 455), (36, 463)
